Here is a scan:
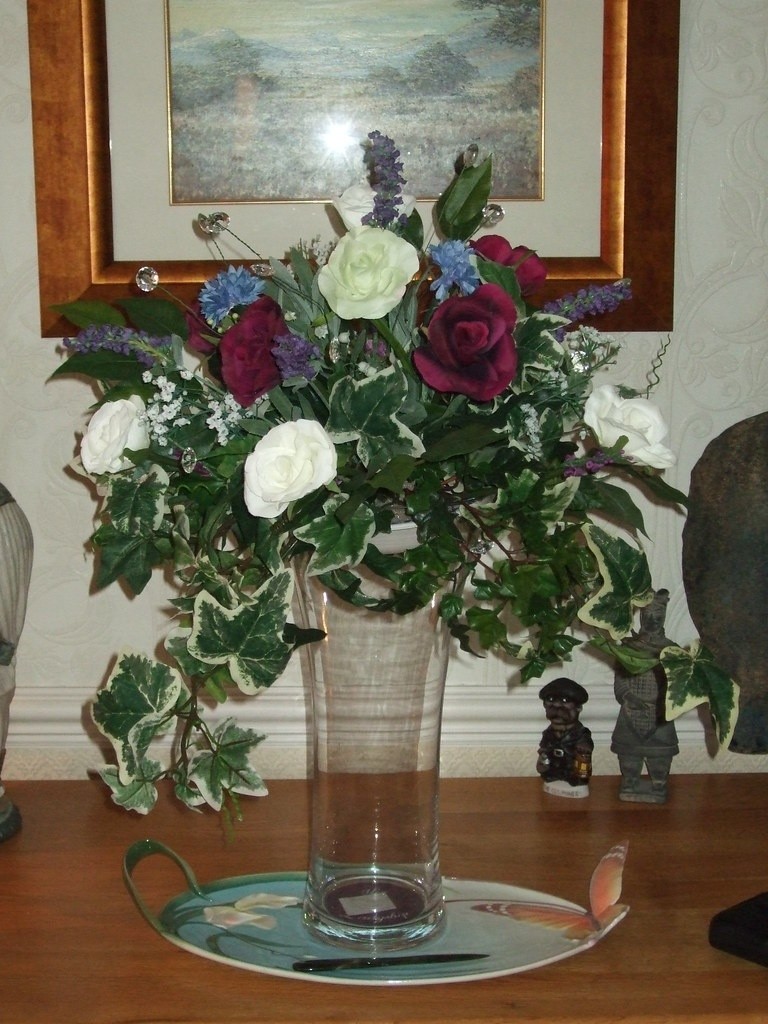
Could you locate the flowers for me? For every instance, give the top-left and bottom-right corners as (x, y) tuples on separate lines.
(42, 130), (742, 849)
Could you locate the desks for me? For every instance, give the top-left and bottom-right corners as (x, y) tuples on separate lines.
(0, 772), (768, 1023)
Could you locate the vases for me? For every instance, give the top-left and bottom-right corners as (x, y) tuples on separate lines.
(291, 528), (480, 950)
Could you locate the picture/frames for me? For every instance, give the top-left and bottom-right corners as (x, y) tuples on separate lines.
(28, 1), (680, 337)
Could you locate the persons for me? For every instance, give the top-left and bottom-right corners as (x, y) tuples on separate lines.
(537, 678), (594, 786)
(611, 589), (681, 792)
